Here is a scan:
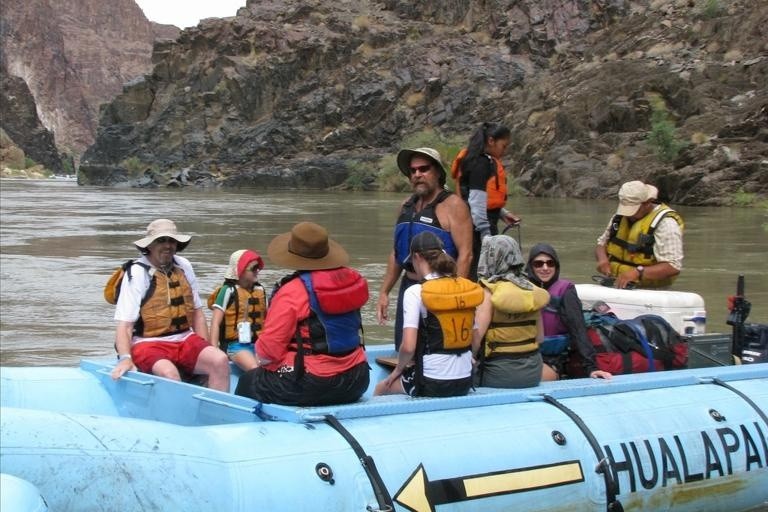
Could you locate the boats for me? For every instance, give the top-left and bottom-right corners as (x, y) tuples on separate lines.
(0, 277), (768, 512)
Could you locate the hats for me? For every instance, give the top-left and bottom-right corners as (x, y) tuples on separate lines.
(224, 246), (266, 281)
(617, 181), (649, 216)
(269, 223), (349, 271)
(134, 220), (192, 253)
(403, 231), (445, 264)
(397, 148), (446, 187)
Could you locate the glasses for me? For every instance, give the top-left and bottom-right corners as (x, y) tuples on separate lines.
(533, 260), (555, 267)
(156, 238), (175, 245)
(408, 162), (435, 173)
(245, 264), (261, 274)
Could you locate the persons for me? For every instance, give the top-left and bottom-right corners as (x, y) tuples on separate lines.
(595, 180), (684, 289)
(210, 249), (268, 372)
(471, 234), (550, 388)
(376, 147), (475, 351)
(374, 231), (485, 398)
(525, 242), (613, 382)
(451, 122), (521, 239)
(235, 222), (370, 407)
(111, 219), (231, 393)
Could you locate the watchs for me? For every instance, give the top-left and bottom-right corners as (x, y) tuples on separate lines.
(636, 265), (646, 282)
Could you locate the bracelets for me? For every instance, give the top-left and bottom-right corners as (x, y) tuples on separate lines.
(118, 353), (133, 359)
